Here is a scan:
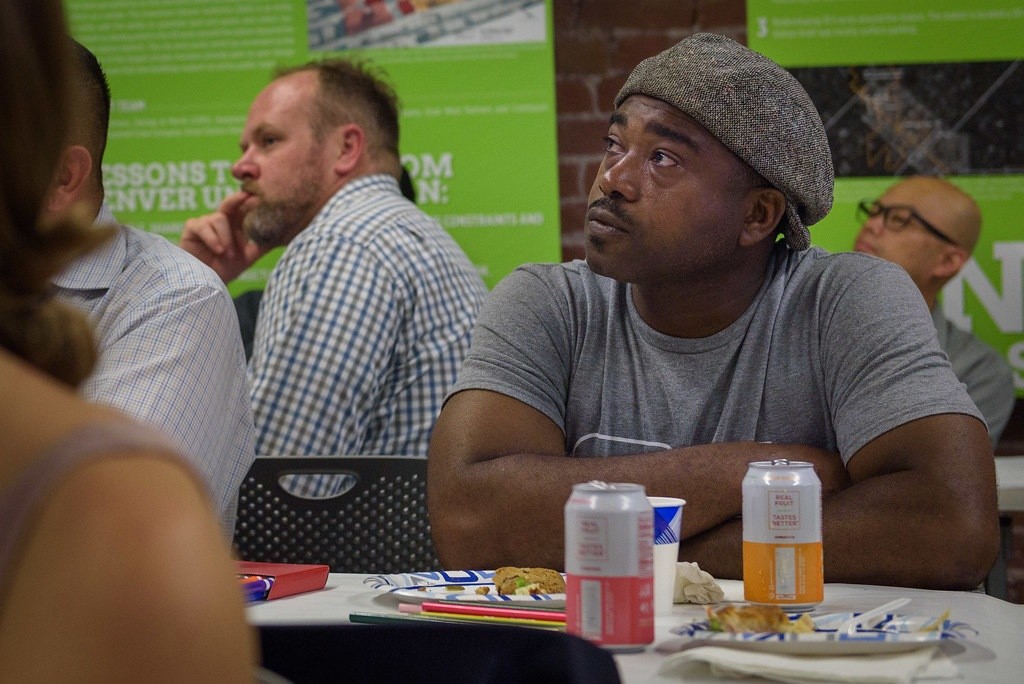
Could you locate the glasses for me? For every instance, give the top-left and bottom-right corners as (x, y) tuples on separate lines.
(855, 198), (957, 246)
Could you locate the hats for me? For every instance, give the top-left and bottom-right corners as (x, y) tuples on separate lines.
(613, 33), (836, 251)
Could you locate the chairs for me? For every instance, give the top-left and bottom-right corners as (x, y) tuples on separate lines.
(231, 453), (443, 575)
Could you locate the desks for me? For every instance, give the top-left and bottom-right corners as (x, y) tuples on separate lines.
(245, 569), (1023, 684)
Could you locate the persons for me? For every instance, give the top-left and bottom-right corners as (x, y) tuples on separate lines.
(178, 56), (488, 497)
(854, 175), (1015, 452)
(1, 0), (252, 683)
(31, 36), (258, 546)
(426, 33), (1003, 590)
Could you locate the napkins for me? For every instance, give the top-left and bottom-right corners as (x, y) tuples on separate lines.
(658, 637), (960, 684)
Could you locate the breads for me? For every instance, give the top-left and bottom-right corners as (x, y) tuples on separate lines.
(706, 603), (790, 634)
(492, 566), (566, 595)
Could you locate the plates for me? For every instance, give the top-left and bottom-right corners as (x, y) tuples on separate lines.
(669, 611), (977, 655)
(363, 570), (567, 604)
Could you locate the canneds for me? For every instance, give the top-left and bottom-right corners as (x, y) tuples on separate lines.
(564, 479), (654, 654)
(741, 456), (824, 613)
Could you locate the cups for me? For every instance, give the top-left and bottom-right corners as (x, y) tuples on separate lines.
(647, 496), (686, 616)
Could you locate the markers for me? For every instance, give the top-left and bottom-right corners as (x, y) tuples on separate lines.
(234, 574), (276, 606)
